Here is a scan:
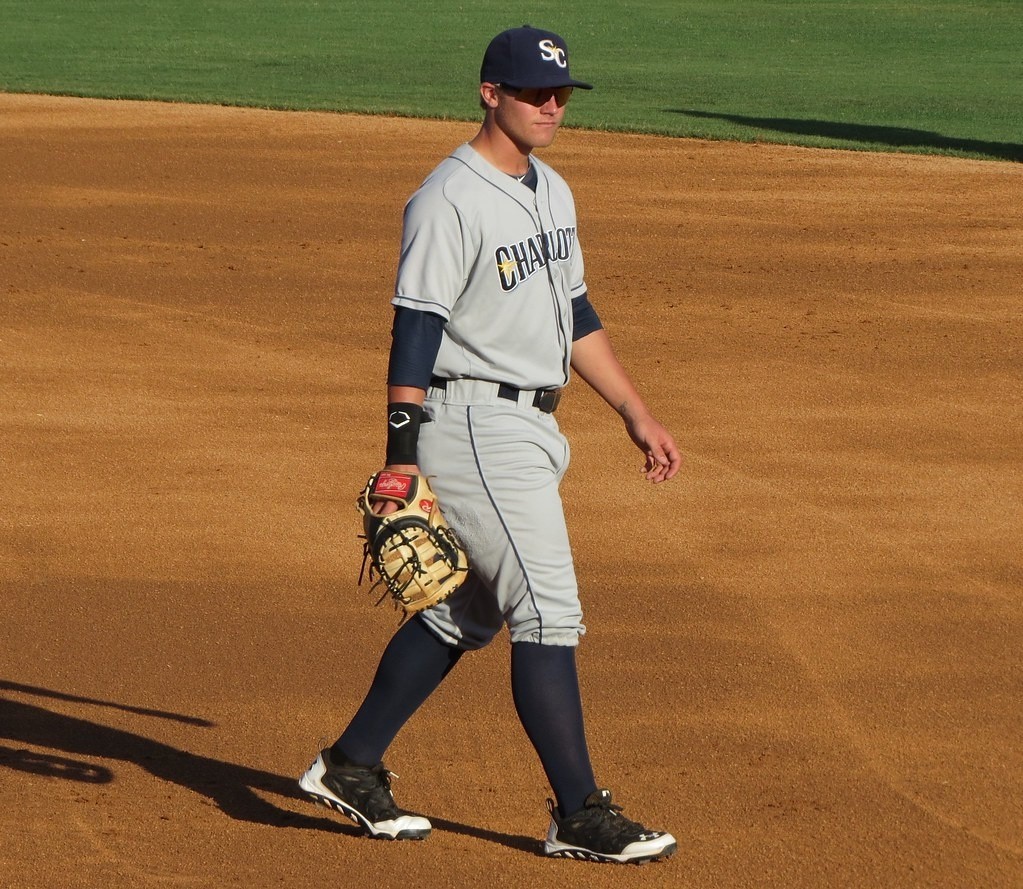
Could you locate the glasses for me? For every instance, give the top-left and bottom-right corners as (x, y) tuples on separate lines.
(495, 83), (573, 107)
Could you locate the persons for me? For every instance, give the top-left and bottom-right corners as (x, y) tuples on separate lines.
(297, 25), (679, 865)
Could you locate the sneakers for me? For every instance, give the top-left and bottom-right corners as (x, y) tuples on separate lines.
(544, 789), (678, 866)
(298, 737), (433, 841)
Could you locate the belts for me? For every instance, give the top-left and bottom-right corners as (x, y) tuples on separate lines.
(429, 375), (561, 413)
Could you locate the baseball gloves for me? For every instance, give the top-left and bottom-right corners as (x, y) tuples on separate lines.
(352, 469), (474, 627)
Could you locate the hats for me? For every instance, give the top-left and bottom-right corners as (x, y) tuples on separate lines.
(480, 24), (594, 91)
